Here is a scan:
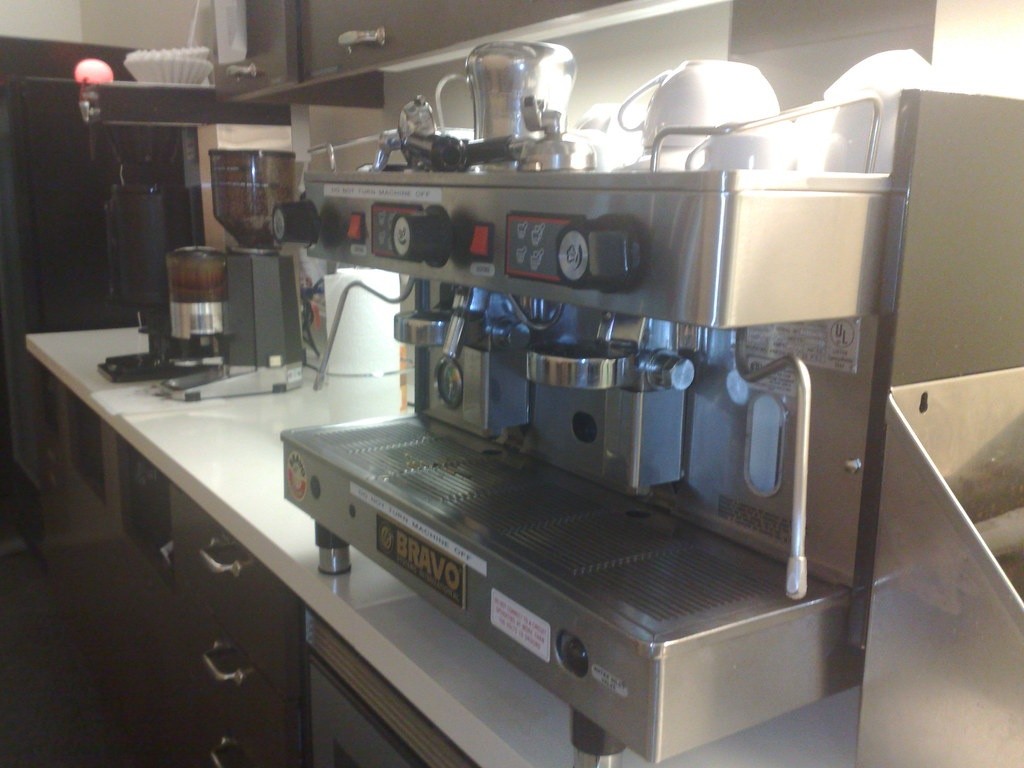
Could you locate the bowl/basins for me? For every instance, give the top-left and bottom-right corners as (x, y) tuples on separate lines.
(124, 47), (213, 84)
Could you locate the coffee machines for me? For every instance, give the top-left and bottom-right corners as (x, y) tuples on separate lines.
(97, 148), (303, 401)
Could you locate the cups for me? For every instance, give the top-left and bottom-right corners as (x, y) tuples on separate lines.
(823, 48), (932, 173)
(686, 134), (774, 171)
(617, 59), (781, 145)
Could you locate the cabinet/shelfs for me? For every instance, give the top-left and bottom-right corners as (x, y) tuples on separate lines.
(170, 482), (302, 768)
(296, 597), (478, 768)
(211, 0), (719, 109)
